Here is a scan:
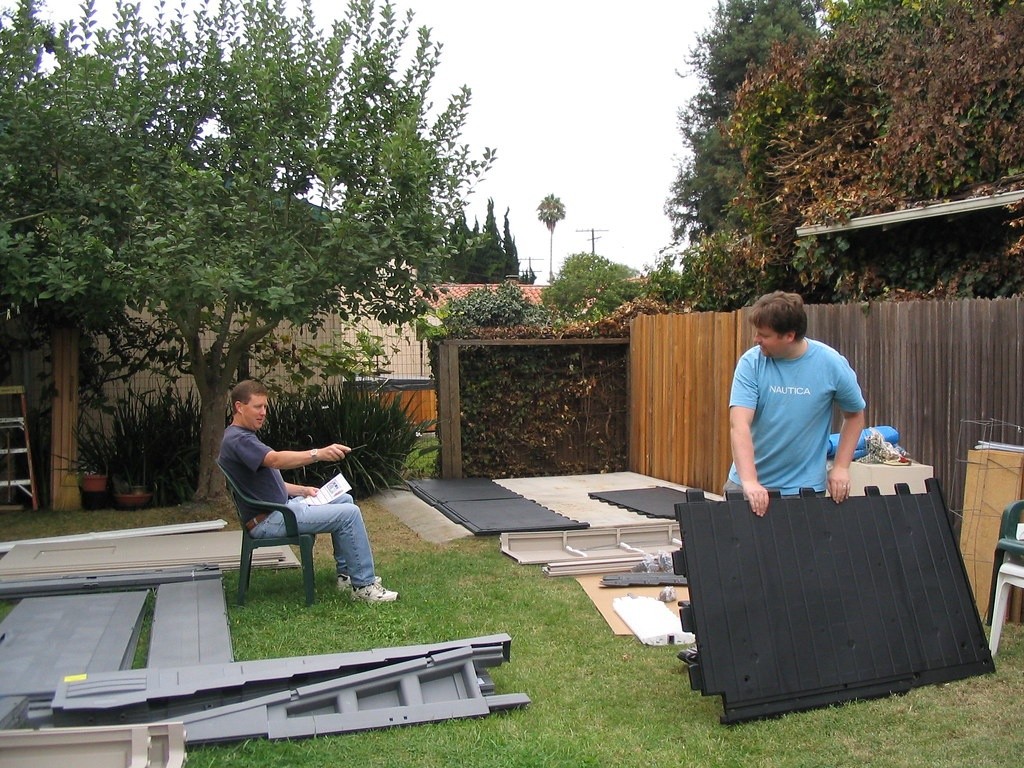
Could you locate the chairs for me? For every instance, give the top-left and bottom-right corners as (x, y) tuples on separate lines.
(985, 500), (1024, 657)
(215, 458), (317, 607)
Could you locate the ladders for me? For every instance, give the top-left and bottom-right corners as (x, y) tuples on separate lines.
(0, 383), (40, 515)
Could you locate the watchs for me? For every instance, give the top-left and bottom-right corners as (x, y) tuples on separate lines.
(310, 449), (318, 462)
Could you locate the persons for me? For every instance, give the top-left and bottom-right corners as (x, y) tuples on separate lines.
(677, 291), (866, 665)
(217, 380), (399, 601)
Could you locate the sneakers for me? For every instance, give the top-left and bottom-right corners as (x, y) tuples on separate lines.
(350, 581), (399, 605)
(337, 573), (382, 590)
(677, 645), (699, 664)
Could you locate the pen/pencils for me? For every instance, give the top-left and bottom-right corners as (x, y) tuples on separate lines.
(344, 444), (367, 454)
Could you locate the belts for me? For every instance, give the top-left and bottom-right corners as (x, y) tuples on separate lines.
(246, 512), (272, 532)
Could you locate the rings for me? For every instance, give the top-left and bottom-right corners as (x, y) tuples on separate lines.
(843, 485), (846, 488)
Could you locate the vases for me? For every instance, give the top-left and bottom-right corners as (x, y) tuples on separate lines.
(81, 474), (108, 492)
